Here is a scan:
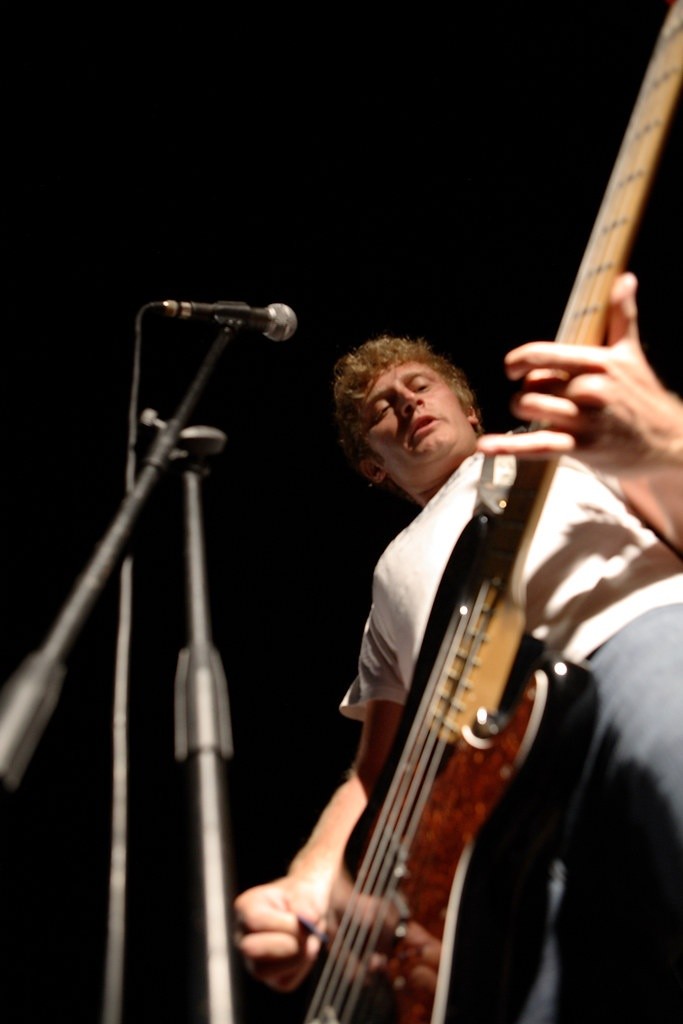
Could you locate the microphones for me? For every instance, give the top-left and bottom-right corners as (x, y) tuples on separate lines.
(148, 300), (297, 342)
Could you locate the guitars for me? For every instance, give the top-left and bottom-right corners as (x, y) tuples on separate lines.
(292, 0), (681, 1024)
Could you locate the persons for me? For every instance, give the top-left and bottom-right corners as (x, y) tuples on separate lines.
(233, 271), (681, 1023)
